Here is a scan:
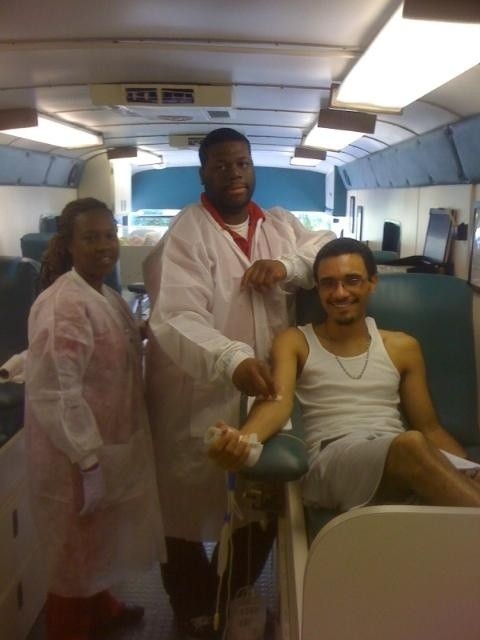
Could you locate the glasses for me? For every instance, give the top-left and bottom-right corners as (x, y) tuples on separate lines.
(318, 276), (369, 290)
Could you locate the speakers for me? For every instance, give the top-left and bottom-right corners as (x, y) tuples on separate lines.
(423, 208), (457, 264)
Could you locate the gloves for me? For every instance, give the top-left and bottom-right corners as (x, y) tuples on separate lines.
(76, 461), (119, 519)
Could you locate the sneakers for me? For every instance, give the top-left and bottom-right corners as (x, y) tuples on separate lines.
(100, 600), (146, 633)
(175, 613), (220, 640)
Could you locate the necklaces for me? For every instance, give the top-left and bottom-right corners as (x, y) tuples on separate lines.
(323, 318), (372, 379)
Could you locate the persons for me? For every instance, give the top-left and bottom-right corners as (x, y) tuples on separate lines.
(137, 128), (340, 640)
(19, 197), (168, 640)
(209, 236), (480, 508)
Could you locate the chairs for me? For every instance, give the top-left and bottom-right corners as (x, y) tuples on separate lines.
(0, 213), (72, 441)
(298, 274), (480, 543)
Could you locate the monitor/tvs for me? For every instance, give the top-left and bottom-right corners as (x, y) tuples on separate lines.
(382, 220), (400, 252)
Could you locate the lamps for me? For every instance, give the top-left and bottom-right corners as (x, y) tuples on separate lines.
(287, 0), (479, 168)
(1, 110), (164, 169)
